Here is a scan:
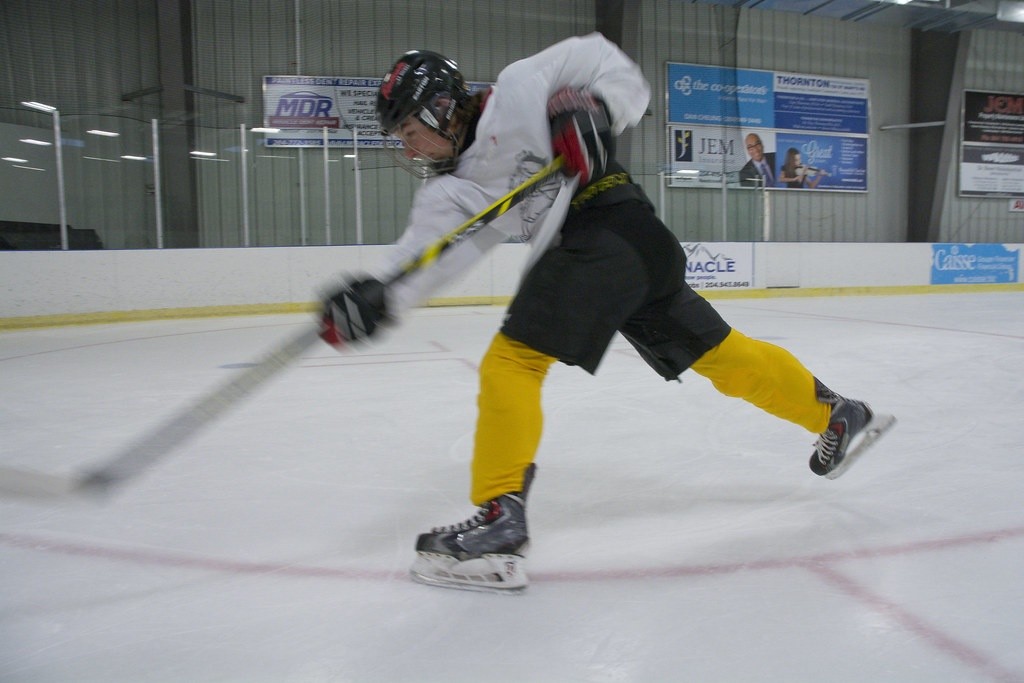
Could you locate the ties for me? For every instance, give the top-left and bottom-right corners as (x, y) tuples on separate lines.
(760, 163), (772, 186)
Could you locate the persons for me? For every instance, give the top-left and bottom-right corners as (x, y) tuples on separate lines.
(739, 133), (776, 187)
(318, 33), (895, 596)
(780, 148), (826, 188)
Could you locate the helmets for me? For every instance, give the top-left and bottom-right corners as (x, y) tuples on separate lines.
(378, 51), (476, 178)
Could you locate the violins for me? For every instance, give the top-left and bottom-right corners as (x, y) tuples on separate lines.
(794, 163), (832, 178)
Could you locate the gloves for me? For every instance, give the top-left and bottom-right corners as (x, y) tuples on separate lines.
(318, 276), (388, 345)
(546, 87), (617, 185)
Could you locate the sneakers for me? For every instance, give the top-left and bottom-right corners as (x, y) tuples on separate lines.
(410, 462), (535, 592)
(808, 377), (896, 480)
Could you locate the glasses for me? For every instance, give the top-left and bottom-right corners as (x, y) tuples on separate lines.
(747, 142), (762, 151)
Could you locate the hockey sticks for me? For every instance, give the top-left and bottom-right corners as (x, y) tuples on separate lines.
(2, 151), (568, 511)
